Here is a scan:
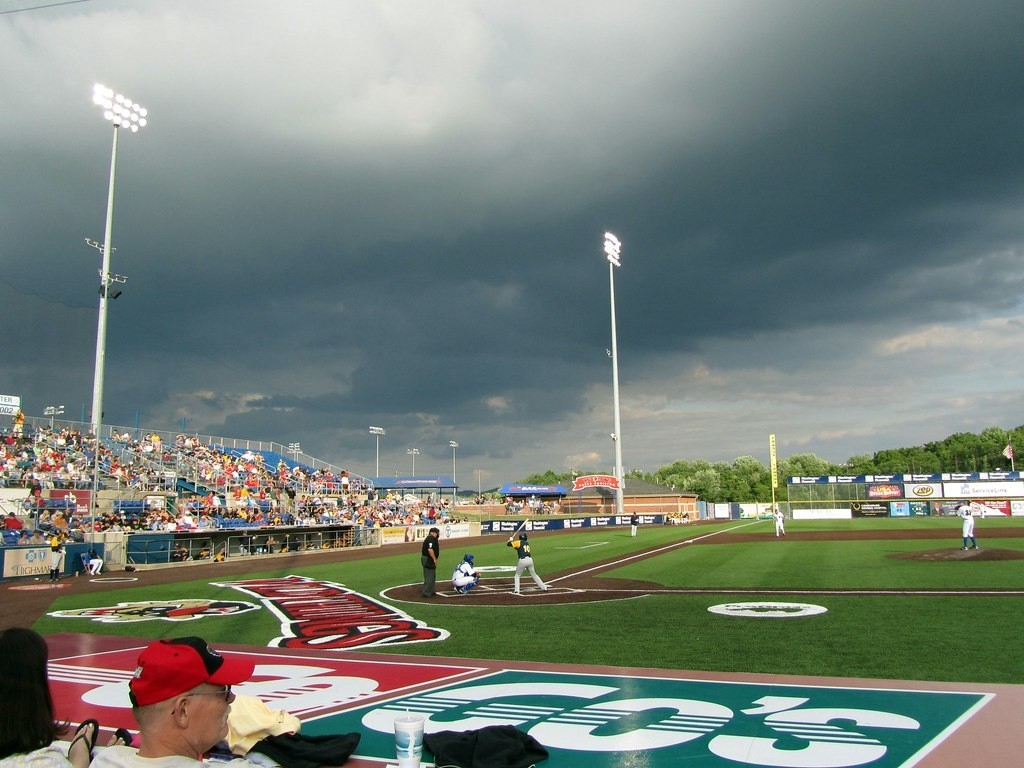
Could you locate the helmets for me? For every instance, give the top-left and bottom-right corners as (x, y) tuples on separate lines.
(464, 554), (475, 569)
(518, 533), (527, 541)
(430, 528), (439, 532)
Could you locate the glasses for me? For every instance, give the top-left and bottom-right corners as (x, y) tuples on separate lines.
(168, 683), (232, 716)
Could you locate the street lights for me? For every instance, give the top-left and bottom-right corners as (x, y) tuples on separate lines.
(601, 231), (626, 516)
(91, 81), (149, 432)
(287, 441), (303, 462)
(368, 426), (483, 501)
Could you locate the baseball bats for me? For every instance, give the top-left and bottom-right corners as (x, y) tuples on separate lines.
(508, 518), (529, 543)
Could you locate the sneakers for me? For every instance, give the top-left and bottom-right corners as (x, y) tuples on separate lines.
(49, 578), (53, 582)
(54, 577), (60, 581)
(453, 586), (467, 595)
(96, 571), (101, 575)
(90, 571), (95, 576)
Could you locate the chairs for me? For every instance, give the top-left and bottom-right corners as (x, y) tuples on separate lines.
(81, 552), (91, 574)
(0, 426), (468, 548)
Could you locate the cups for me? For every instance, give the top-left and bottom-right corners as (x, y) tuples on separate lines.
(394, 711), (425, 768)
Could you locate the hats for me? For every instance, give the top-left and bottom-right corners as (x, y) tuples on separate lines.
(129, 635), (256, 708)
(0, 485), (255, 546)
(89, 548), (95, 556)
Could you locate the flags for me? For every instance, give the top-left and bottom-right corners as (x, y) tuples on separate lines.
(1002, 444), (1015, 460)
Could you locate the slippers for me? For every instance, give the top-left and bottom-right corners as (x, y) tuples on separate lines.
(67, 718), (99, 764)
(107, 728), (133, 747)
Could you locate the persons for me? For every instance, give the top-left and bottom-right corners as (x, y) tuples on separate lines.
(451, 554), (482, 594)
(772, 509), (785, 538)
(1, 628), (132, 768)
(666, 511), (690, 524)
(0, 410), (561, 562)
(84, 548), (104, 576)
(507, 533), (548, 595)
(954, 500), (978, 551)
(979, 502), (986, 520)
(49, 530), (63, 581)
(422, 528), (440, 598)
(631, 511), (639, 537)
(88, 636), (267, 768)
(931, 502), (944, 516)
